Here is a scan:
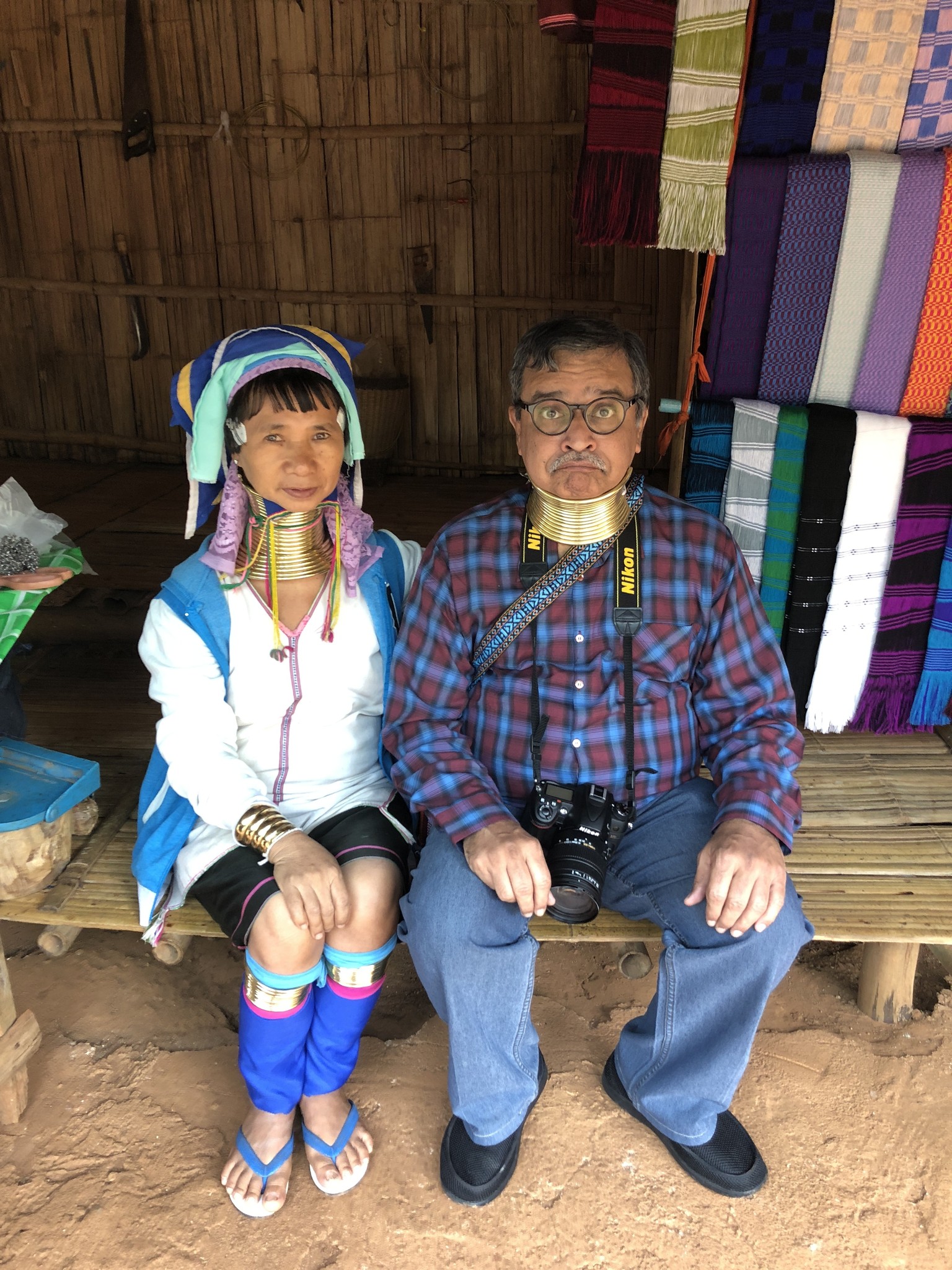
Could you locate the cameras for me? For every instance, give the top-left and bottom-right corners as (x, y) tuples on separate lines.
(519, 779), (633, 923)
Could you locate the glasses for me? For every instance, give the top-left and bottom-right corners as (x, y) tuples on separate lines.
(513, 394), (640, 434)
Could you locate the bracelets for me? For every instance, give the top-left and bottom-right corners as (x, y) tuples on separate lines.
(235, 804), (301, 862)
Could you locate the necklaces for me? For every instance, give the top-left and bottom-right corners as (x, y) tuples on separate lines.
(527, 468), (636, 545)
(231, 479), (339, 582)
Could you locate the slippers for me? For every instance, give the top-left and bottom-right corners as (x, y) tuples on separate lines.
(302, 1094), (373, 1194)
(225, 1124), (293, 1217)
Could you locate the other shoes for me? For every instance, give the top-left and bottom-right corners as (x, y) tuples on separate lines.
(439, 1047), (547, 1206)
(602, 1050), (768, 1198)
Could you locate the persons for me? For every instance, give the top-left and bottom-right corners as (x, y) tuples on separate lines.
(131, 325), (430, 1220)
(379, 312), (818, 1207)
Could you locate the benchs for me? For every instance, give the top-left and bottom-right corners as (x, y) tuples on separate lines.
(1, 732), (952, 1023)
